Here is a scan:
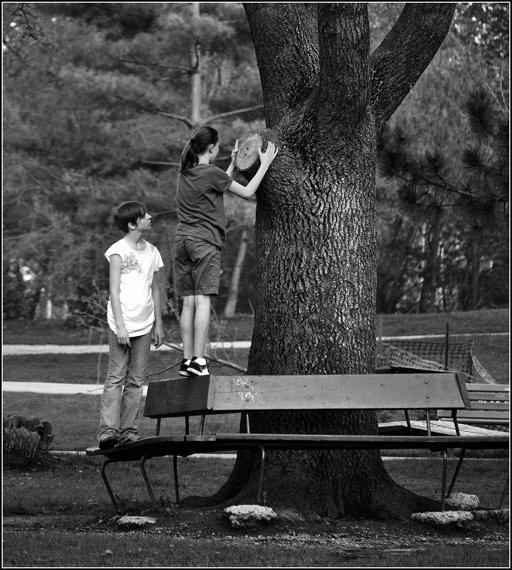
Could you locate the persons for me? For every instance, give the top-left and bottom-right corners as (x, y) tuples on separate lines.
(172, 126), (280, 379)
(95, 201), (165, 448)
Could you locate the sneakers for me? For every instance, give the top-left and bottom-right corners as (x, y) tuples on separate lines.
(98, 434), (118, 449)
(178, 358), (192, 377)
(186, 356), (210, 376)
(119, 433), (140, 445)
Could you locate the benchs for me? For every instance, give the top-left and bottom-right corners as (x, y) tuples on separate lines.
(207, 374), (510, 511)
(375, 419), (510, 511)
(437, 382), (509, 432)
(85, 376), (216, 515)
